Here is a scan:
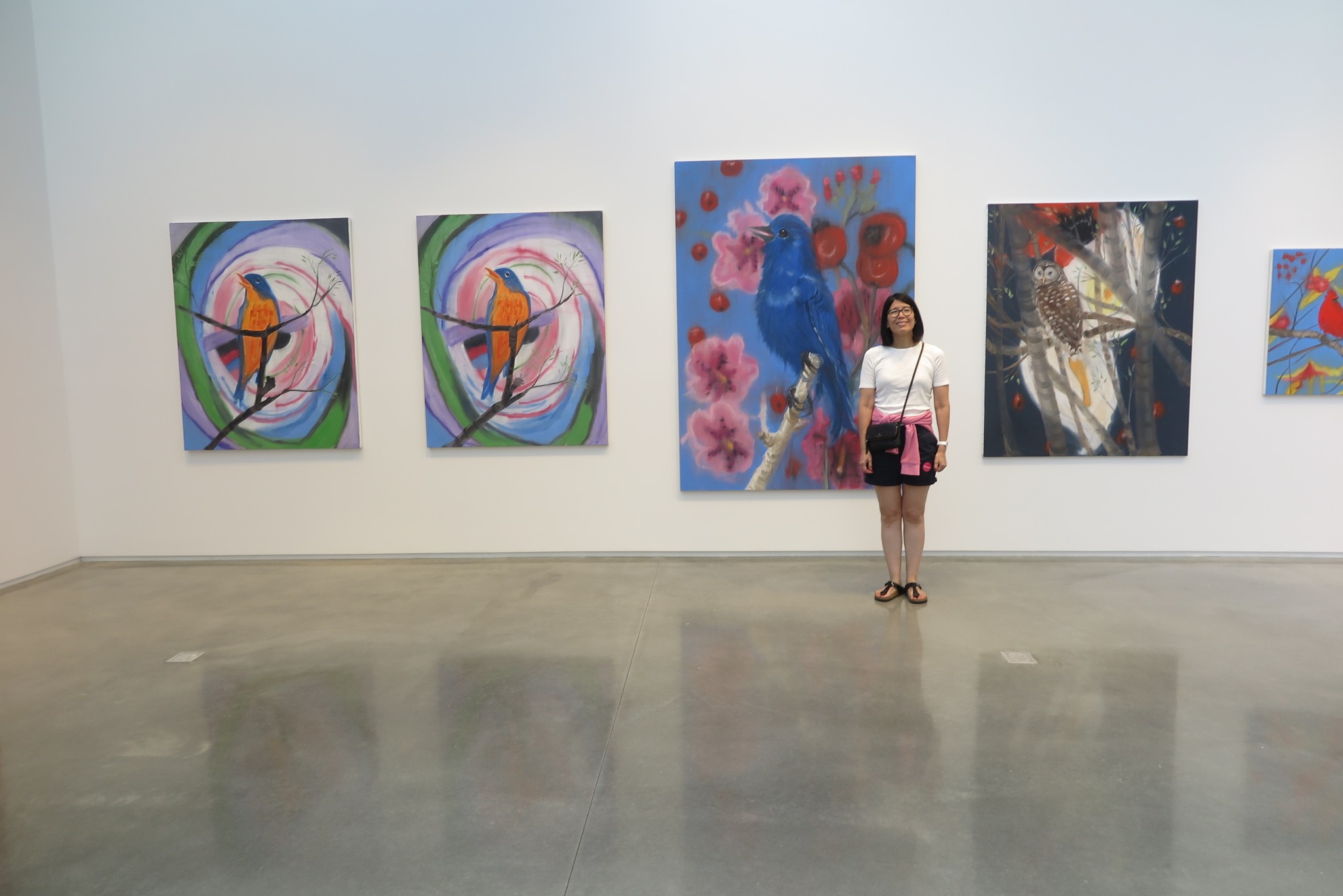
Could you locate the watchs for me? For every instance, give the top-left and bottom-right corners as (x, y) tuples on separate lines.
(936, 440), (948, 447)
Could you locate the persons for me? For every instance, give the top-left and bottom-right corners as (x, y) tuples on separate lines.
(858, 292), (950, 604)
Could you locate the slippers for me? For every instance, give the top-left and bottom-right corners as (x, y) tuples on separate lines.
(904, 582), (928, 604)
(874, 580), (904, 601)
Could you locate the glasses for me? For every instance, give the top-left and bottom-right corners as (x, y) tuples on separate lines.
(888, 306), (913, 318)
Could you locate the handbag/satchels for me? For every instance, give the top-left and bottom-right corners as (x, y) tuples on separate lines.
(865, 422), (901, 452)
